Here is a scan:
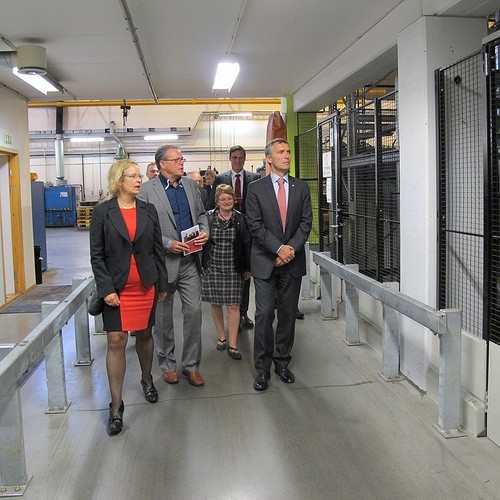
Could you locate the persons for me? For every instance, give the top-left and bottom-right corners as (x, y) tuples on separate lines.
(129, 162), (160, 337)
(88, 160), (168, 435)
(191, 173), (209, 211)
(200, 183), (250, 359)
(246, 137), (313, 390)
(204, 169), (217, 196)
(137, 145), (210, 385)
(214, 168), (218, 174)
(275, 297), (305, 319)
(207, 166), (211, 170)
(214, 145), (262, 334)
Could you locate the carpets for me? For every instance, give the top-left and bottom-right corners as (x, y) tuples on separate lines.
(0, 284), (74, 314)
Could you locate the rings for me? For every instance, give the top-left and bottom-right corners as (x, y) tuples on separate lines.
(107, 303), (112, 306)
(288, 259), (290, 261)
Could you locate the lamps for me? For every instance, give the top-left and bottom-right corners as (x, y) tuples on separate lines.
(210, 52), (238, 95)
(12, 64), (62, 96)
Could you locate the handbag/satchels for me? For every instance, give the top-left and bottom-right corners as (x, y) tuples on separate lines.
(88, 290), (104, 316)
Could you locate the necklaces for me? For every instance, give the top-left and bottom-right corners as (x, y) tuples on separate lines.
(116, 197), (136, 209)
(219, 209), (226, 221)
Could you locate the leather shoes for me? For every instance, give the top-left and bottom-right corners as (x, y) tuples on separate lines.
(254, 370), (271, 391)
(163, 369), (179, 383)
(181, 369), (204, 386)
(275, 368), (295, 383)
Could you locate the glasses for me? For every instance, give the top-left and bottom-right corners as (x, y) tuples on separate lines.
(122, 174), (144, 179)
(163, 158), (186, 164)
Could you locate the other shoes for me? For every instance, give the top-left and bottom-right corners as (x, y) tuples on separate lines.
(108, 399), (124, 435)
(227, 344), (241, 360)
(296, 309), (304, 317)
(241, 313), (253, 327)
(216, 336), (226, 351)
(141, 378), (158, 403)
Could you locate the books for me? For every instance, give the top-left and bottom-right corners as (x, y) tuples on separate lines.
(182, 224), (203, 256)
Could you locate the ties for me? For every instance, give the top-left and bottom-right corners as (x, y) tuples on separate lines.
(235, 174), (242, 211)
(277, 178), (287, 234)
